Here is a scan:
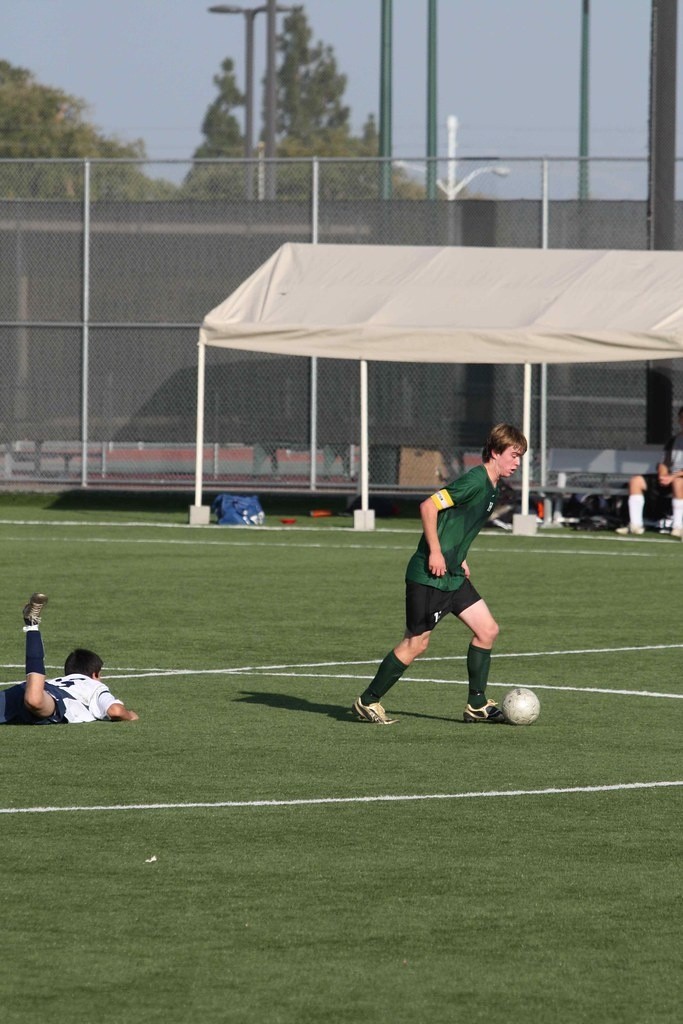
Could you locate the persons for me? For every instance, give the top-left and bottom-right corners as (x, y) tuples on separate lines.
(615, 406), (683, 538)
(0, 593), (139, 725)
(350, 423), (528, 724)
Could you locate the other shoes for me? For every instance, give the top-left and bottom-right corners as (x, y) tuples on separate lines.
(617, 525), (645, 535)
(671, 527), (682, 539)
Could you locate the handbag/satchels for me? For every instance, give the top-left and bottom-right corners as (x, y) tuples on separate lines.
(213, 494), (265, 526)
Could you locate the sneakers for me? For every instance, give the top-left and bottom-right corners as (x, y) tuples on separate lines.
(351, 696), (399, 724)
(24, 593), (48, 626)
(463, 699), (508, 724)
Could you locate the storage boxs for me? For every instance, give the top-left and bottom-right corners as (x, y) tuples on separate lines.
(399, 446), (441, 486)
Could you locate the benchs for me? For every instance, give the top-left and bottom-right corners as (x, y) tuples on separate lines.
(510, 448), (663, 530)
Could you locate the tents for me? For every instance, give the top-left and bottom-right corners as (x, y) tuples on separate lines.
(194, 242), (683, 505)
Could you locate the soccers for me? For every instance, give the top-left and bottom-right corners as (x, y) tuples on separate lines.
(503, 687), (542, 726)
